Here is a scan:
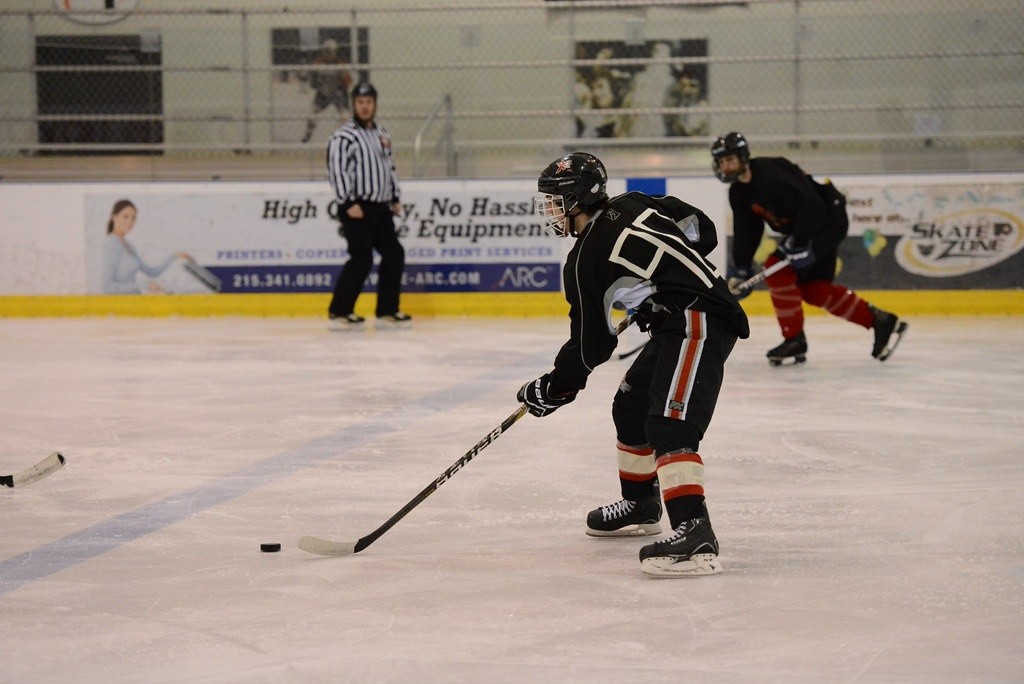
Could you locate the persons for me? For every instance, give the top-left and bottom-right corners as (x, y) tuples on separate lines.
(327, 82), (413, 333)
(573, 41), (710, 137)
(710, 131), (908, 366)
(517, 151), (750, 578)
(300, 39), (356, 142)
(98, 199), (195, 295)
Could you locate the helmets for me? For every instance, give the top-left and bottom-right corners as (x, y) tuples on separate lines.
(710, 132), (750, 183)
(534, 151), (609, 237)
(351, 83), (378, 98)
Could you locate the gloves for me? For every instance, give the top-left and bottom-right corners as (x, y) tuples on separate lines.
(516, 372), (579, 418)
(729, 265), (754, 300)
(779, 233), (811, 256)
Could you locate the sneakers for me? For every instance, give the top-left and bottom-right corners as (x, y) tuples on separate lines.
(767, 330), (808, 366)
(374, 309), (413, 329)
(871, 303), (907, 362)
(586, 480), (666, 538)
(637, 510), (722, 576)
(327, 311), (369, 332)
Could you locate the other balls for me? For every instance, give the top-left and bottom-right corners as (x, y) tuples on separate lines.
(260, 543), (282, 552)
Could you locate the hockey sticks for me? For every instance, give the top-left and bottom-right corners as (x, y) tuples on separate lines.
(609, 259), (791, 362)
(294, 311), (639, 558)
(0, 450), (71, 492)
